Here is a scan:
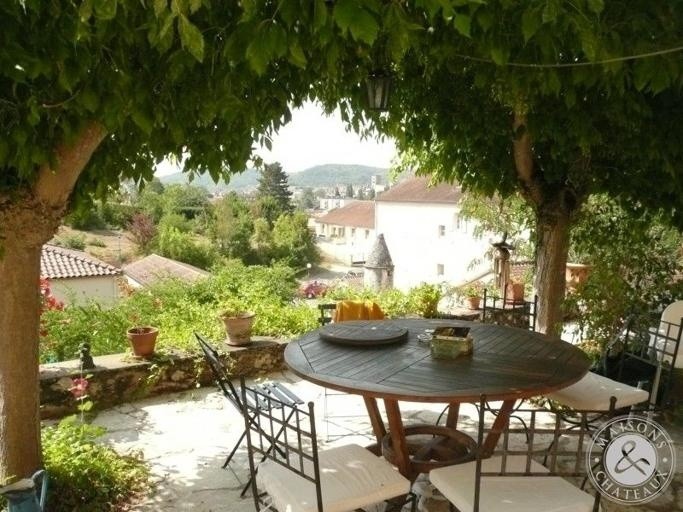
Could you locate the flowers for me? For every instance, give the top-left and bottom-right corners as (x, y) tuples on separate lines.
(118, 275), (164, 332)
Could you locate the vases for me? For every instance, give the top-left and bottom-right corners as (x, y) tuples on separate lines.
(127, 326), (159, 356)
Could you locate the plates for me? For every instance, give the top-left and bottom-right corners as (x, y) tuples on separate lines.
(317, 320), (409, 344)
